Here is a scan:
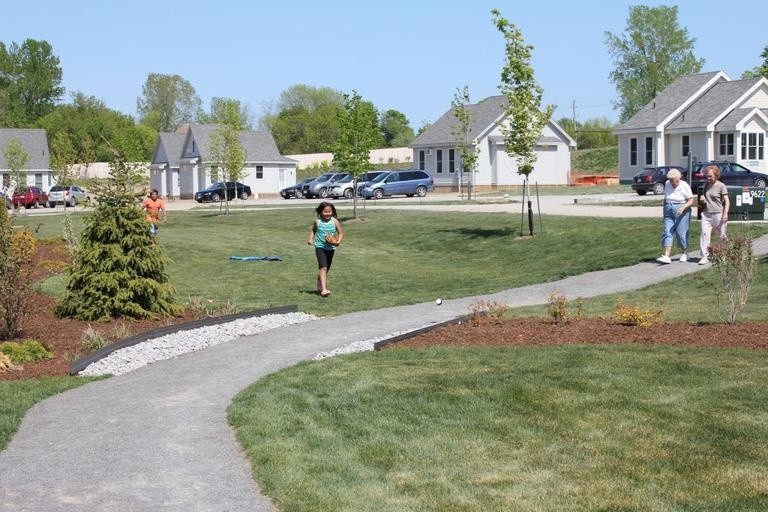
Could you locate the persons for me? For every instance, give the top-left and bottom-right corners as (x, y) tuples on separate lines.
(697, 164), (731, 265)
(140, 189), (167, 237)
(655, 168), (694, 264)
(306, 202), (344, 297)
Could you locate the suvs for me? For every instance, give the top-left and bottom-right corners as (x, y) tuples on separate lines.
(630, 165), (686, 195)
(300, 171), (347, 200)
(11, 184), (49, 210)
(45, 185), (90, 208)
(684, 160), (768, 194)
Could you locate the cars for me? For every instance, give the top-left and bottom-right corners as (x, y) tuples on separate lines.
(280, 177), (316, 199)
(194, 181), (252, 204)
(0, 191), (11, 210)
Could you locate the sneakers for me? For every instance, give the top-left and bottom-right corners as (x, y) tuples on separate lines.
(656, 254), (671, 264)
(699, 257), (710, 265)
(679, 254), (689, 262)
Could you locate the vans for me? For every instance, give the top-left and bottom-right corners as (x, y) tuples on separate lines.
(362, 169), (434, 199)
(327, 171), (392, 199)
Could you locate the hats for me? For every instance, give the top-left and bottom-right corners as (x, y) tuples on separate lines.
(667, 168), (681, 179)
